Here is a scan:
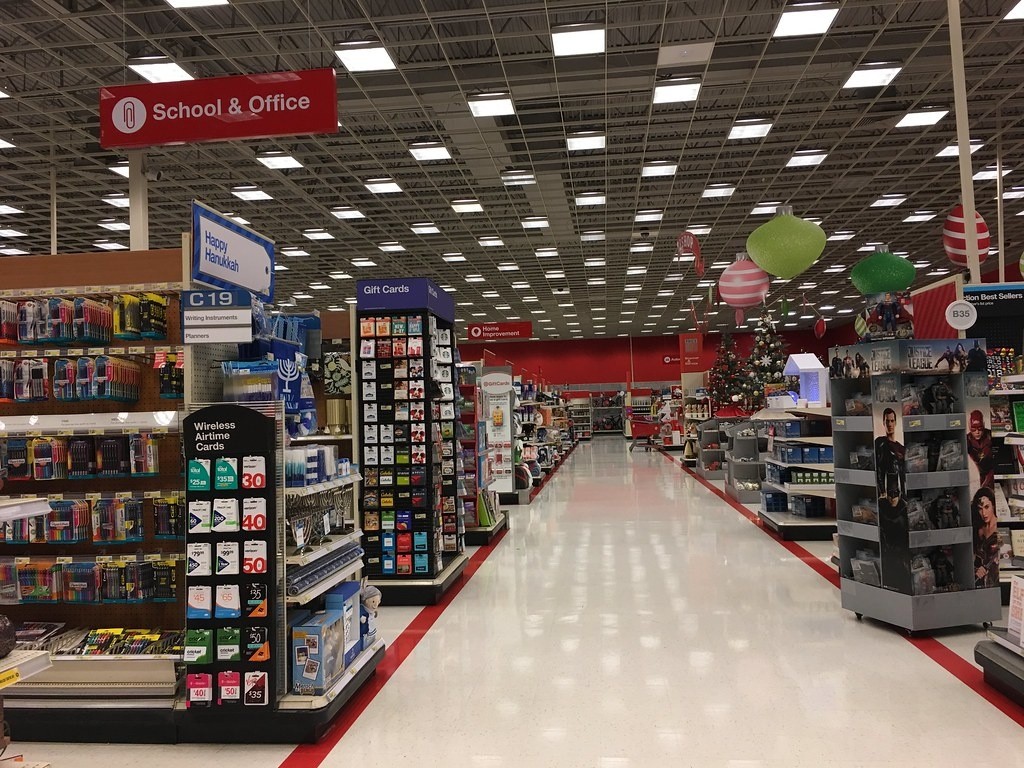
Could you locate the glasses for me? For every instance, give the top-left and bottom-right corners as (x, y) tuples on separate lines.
(970, 427), (984, 432)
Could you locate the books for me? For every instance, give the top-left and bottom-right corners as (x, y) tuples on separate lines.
(990, 397), (1022, 568)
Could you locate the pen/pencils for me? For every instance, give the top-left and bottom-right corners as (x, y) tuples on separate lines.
(1, 294), (184, 657)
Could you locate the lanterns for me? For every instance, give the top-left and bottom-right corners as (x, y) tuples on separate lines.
(746, 214), (826, 280)
(850, 253), (915, 296)
(718, 262), (770, 308)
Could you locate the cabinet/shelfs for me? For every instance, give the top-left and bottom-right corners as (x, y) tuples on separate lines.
(0, 236), (1024, 744)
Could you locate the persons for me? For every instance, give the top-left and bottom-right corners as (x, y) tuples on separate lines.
(965, 409), (994, 488)
(933, 340), (986, 373)
(877, 490), (911, 594)
(970, 489), (1000, 589)
(874, 406), (905, 497)
(830, 347), (869, 378)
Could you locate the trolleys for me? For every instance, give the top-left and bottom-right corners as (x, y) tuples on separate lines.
(628, 415), (663, 453)
